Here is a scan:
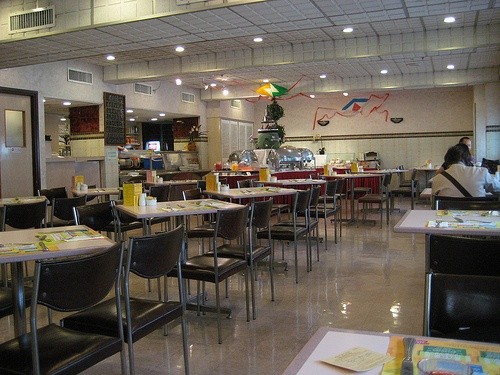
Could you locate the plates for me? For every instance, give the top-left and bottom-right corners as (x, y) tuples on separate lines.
(178, 163), (200, 171)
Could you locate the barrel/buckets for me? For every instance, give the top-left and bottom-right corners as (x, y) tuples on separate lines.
(256, 129), (280, 148)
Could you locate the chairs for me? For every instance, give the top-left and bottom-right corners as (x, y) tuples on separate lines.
(0, 158), (499, 375)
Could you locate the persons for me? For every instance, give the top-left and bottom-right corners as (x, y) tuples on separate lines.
(436, 137), (471, 174)
(432, 144), (500, 198)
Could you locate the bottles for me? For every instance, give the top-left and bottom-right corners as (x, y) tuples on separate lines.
(214, 160), (251, 171)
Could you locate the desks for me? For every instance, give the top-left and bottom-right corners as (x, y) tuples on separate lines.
(253, 179), (327, 244)
(351, 169), (409, 214)
(409, 166), (441, 188)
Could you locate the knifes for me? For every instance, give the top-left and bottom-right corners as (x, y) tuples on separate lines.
(400, 335), (417, 375)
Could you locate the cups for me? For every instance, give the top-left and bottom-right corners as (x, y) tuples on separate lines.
(76, 183), (88, 191)
(357, 167), (364, 172)
(137, 193), (156, 207)
(417, 358), (472, 375)
(328, 169), (337, 175)
(153, 176), (163, 183)
(270, 176), (277, 181)
(216, 182), (229, 192)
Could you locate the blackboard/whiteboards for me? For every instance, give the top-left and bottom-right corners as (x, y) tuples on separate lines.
(104, 92), (126, 146)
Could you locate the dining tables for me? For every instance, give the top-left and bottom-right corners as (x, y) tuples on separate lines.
(202, 180), (302, 263)
(0, 224), (119, 375)
(115, 196), (245, 305)
(394, 194), (500, 237)
(319, 174), (384, 228)
(284, 325), (500, 374)
